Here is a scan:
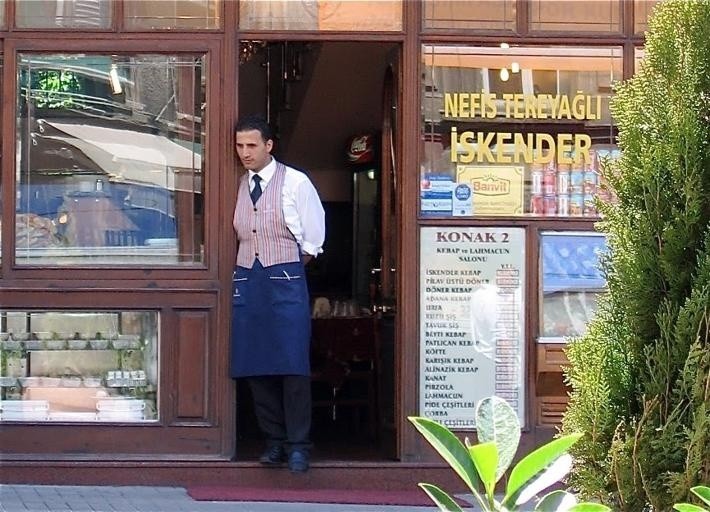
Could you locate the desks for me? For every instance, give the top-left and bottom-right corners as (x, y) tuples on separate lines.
(310, 314), (380, 436)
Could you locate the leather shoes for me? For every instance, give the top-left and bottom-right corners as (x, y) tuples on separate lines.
(259, 445), (286, 465)
(288, 449), (308, 473)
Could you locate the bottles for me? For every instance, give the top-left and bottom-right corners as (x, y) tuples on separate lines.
(103, 228), (150, 245)
(94, 178), (104, 194)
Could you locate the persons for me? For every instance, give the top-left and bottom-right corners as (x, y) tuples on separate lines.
(230, 112), (326, 474)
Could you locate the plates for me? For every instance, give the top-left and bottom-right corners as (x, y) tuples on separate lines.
(143, 237), (179, 245)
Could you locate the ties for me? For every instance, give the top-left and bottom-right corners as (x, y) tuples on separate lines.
(250, 174), (262, 204)
